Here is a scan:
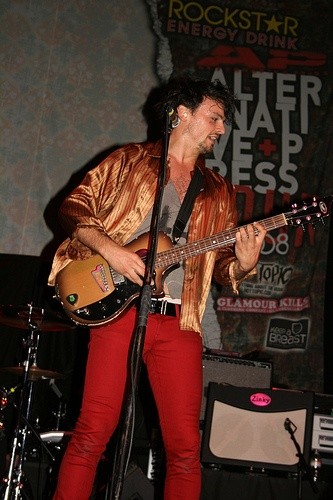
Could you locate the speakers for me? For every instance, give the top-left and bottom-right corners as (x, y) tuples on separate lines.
(200, 381), (315, 480)
(199, 353), (273, 429)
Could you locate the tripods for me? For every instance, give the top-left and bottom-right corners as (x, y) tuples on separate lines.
(0, 323), (68, 500)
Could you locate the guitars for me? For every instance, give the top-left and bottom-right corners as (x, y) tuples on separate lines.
(52, 195), (330, 329)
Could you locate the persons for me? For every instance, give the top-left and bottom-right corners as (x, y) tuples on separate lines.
(52, 80), (266, 499)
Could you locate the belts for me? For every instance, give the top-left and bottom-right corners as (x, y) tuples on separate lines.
(135, 298), (181, 317)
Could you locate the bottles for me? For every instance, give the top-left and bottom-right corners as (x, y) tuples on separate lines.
(310, 449), (321, 488)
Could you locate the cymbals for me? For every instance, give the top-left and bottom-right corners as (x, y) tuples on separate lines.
(0, 304), (77, 334)
(0, 366), (68, 382)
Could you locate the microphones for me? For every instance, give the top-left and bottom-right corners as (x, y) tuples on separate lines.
(165, 106), (181, 128)
